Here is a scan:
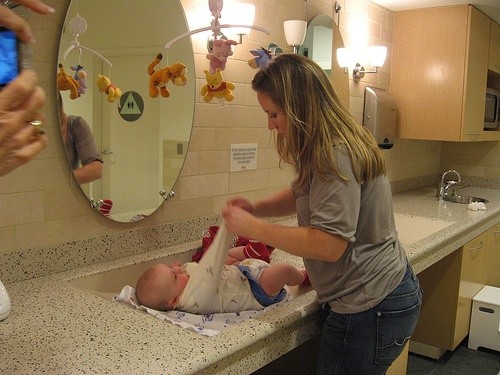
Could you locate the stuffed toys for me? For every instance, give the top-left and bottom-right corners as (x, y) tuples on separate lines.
(147, 53), (187, 98)
(57, 64), (78, 99)
(200, 69), (235, 103)
(247, 47), (270, 69)
(74, 69), (88, 96)
(205, 38), (237, 75)
(96, 74), (123, 104)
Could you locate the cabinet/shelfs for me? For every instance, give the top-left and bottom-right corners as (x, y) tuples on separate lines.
(409, 222), (500, 350)
(390, 5), (500, 143)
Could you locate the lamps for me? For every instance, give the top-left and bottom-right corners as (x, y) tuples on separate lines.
(225, 3), (255, 44)
(283, 20), (307, 54)
(337, 48), (350, 73)
(353, 46), (387, 82)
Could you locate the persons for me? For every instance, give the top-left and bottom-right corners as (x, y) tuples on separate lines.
(59, 92), (103, 185)
(222, 55), (422, 375)
(0, 0), (57, 179)
(135, 207), (316, 314)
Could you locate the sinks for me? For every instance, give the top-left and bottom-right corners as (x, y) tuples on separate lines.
(443, 195), (487, 204)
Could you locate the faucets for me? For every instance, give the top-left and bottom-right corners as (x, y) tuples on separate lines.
(436, 169), (461, 198)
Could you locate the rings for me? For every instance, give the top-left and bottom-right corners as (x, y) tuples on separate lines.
(28, 120), (45, 135)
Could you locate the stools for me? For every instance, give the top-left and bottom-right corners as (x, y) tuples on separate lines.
(468, 285), (500, 351)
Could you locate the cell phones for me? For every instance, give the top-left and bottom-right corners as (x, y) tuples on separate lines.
(0, 26), (22, 88)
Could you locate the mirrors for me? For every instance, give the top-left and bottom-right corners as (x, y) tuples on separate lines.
(56, 0), (196, 223)
(297, 15), (350, 116)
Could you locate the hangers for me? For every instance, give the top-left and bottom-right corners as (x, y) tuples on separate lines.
(60, 13), (113, 69)
(166, 0), (271, 50)
